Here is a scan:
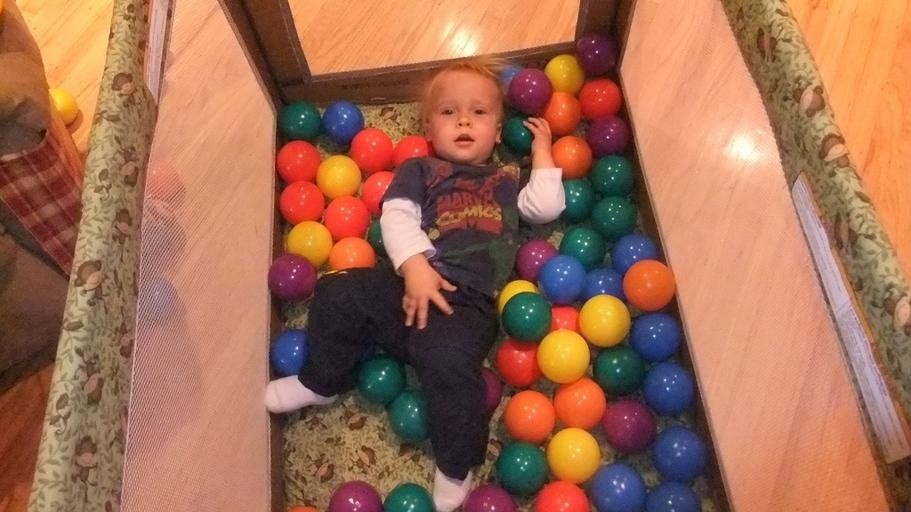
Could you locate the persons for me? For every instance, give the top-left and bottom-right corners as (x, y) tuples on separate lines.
(264, 54), (569, 512)
